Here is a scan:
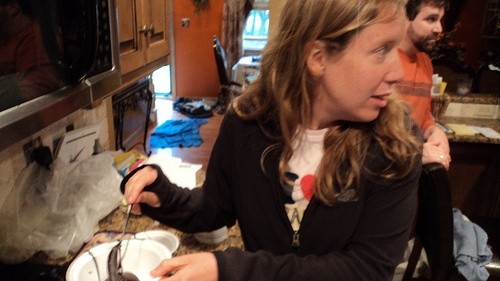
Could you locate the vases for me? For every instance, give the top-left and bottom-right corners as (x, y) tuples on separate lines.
(455, 73), (472, 96)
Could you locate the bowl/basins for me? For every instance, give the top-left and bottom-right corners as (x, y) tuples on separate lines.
(131, 230), (180, 253)
(65, 238), (172, 281)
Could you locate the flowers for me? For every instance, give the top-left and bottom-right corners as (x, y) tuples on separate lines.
(439, 41), (472, 74)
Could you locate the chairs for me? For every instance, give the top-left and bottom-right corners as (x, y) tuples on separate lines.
(415, 162), (468, 281)
(212, 34), (243, 114)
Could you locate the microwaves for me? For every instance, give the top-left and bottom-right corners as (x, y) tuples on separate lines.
(0, 0), (123, 152)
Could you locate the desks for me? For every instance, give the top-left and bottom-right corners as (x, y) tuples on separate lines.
(444, 118), (500, 144)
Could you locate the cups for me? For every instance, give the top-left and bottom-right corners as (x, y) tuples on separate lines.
(194, 225), (228, 244)
(457, 76), (473, 96)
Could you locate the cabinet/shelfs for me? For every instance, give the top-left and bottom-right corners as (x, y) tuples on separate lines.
(448, 141), (500, 258)
(115, 0), (171, 92)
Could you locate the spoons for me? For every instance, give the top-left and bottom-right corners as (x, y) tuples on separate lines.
(108, 203), (132, 281)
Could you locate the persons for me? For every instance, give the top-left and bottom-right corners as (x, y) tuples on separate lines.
(120, 0), (423, 281)
(397, 0), (469, 281)
